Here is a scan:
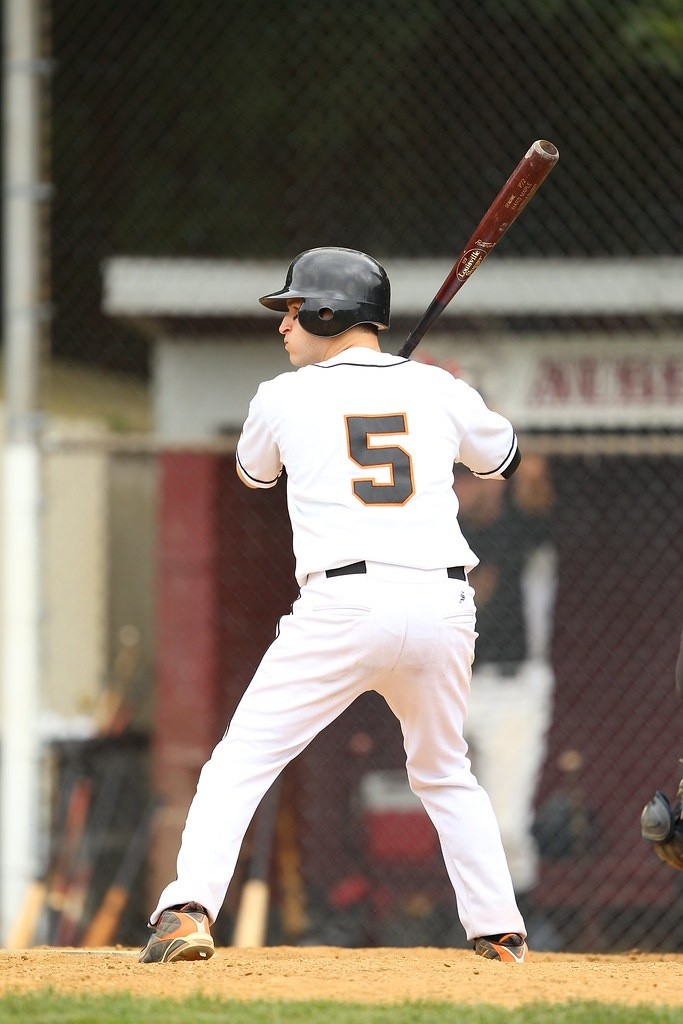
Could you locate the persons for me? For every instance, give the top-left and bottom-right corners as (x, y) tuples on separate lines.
(139, 246), (527, 963)
(450, 454), (558, 944)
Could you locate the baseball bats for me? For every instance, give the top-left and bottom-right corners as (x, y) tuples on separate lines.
(396, 136), (560, 361)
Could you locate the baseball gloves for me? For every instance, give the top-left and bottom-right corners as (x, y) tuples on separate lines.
(639, 776), (683, 871)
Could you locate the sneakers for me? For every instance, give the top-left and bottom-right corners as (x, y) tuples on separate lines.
(474, 930), (529, 965)
(138, 901), (216, 963)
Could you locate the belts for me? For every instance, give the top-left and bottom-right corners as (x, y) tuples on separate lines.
(326, 559), (466, 582)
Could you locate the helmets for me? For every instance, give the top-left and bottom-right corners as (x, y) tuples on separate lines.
(258, 246), (390, 338)
